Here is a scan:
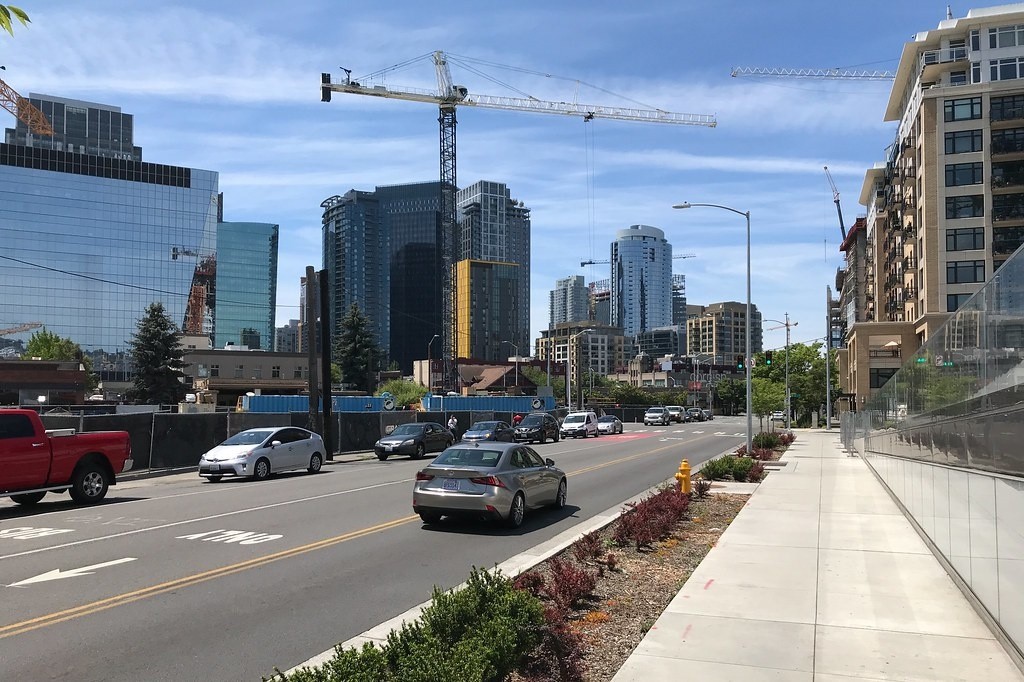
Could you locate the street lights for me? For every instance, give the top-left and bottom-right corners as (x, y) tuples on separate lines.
(428, 335), (440, 391)
(672, 203), (753, 457)
(761, 312), (790, 428)
(501, 341), (518, 386)
(567, 329), (595, 415)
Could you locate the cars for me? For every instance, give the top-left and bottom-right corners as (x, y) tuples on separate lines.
(412, 442), (567, 529)
(198, 427), (326, 482)
(597, 415), (623, 435)
(375, 422), (516, 461)
(771, 411), (784, 421)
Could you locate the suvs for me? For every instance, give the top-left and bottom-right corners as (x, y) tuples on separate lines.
(514, 412), (598, 444)
(644, 406), (713, 426)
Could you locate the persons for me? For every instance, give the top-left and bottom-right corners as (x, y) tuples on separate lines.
(514, 413), (522, 426)
(448, 414), (457, 442)
(426, 425), (432, 433)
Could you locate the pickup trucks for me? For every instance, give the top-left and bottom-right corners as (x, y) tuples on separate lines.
(0, 408), (133, 505)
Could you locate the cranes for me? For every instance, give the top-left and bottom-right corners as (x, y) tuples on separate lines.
(729, 65), (895, 83)
(0, 79), (55, 134)
(320, 50), (719, 396)
(580, 254), (696, 267)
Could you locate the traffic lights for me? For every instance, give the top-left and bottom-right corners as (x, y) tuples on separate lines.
(766, 351), (772, 365)
(738, 356), (743, 369)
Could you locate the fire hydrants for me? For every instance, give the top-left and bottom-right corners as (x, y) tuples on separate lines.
(674, 459), (691, 493)
